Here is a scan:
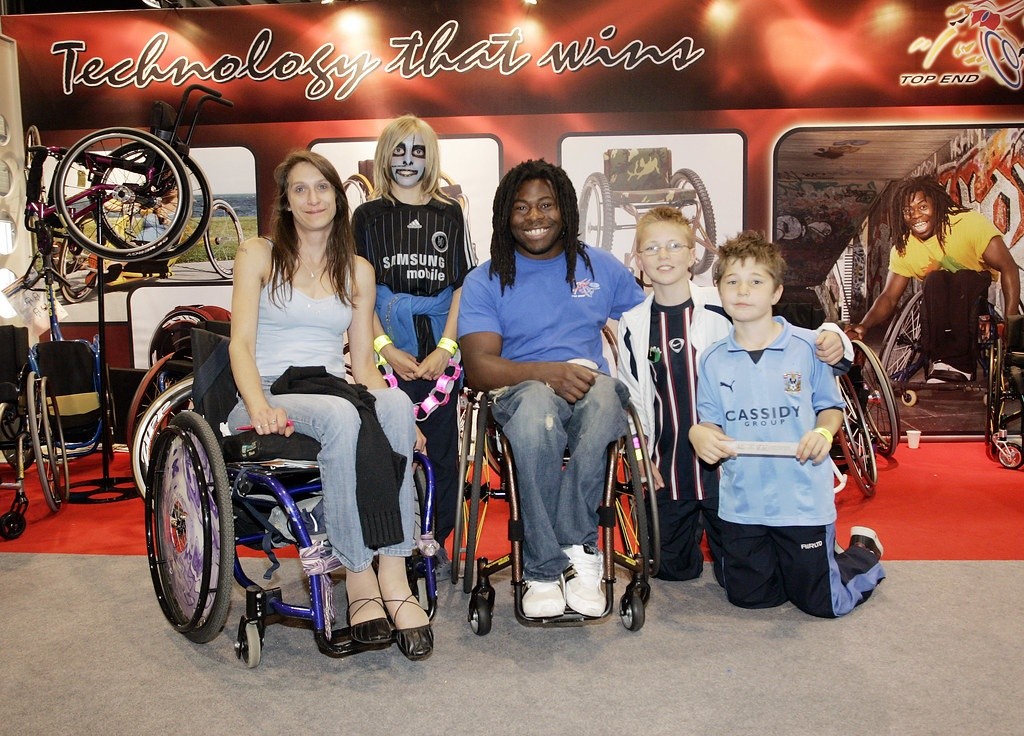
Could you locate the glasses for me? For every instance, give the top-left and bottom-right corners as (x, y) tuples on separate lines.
(639, 241), (690, 256)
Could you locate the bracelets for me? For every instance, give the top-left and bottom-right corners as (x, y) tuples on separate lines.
(373, 335), (393, 354)
(633, 437), (640, 448)
(437, 337), (458, 357)
(813, 428), (834, 444)
(635, 449), (643, 461)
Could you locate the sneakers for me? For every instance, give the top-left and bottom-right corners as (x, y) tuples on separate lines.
(562, 544), (607, 617)
(834, 539), (845, 556)
(849, 526), (883, 560)
(523, 580), (566, 616)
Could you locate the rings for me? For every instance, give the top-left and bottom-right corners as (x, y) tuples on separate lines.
(262, 424), (269, 427)
(256, 425), (261, 429)
(269, 420), (276, 425)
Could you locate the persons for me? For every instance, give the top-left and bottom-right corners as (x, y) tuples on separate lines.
(842, 175), (1020, 383)
(228, 149), (434, 660)
(616, 207), (856, 589)
(111, 184), (178, 274)
(350, 114), (478, 581)
(457, 158), (647, 617)
(687, 228), (887, 619)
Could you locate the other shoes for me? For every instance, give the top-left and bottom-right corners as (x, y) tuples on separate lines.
(348, 597), (391, 644)
(435, 559), (451, 580)
(378, 594), (434, 661)
(927, 362), (971, 383)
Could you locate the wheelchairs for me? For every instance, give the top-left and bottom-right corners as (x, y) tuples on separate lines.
(986, 314), (1024, 470)
(126, 316), (434, 670)
(831, 338), (900, 498)
(0, 364), (73, 541)
(874, 278), (1008, 404)
(578, 148), (718, 275)
(11, 85), (235, 295)
(340, 157), (482, 269)
(449, 376), (663, 637)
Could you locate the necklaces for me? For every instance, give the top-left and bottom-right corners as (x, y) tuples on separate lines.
(298, 255), (326, 278)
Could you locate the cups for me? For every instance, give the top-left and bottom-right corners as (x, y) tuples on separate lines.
(906, 430), (922, 449)
